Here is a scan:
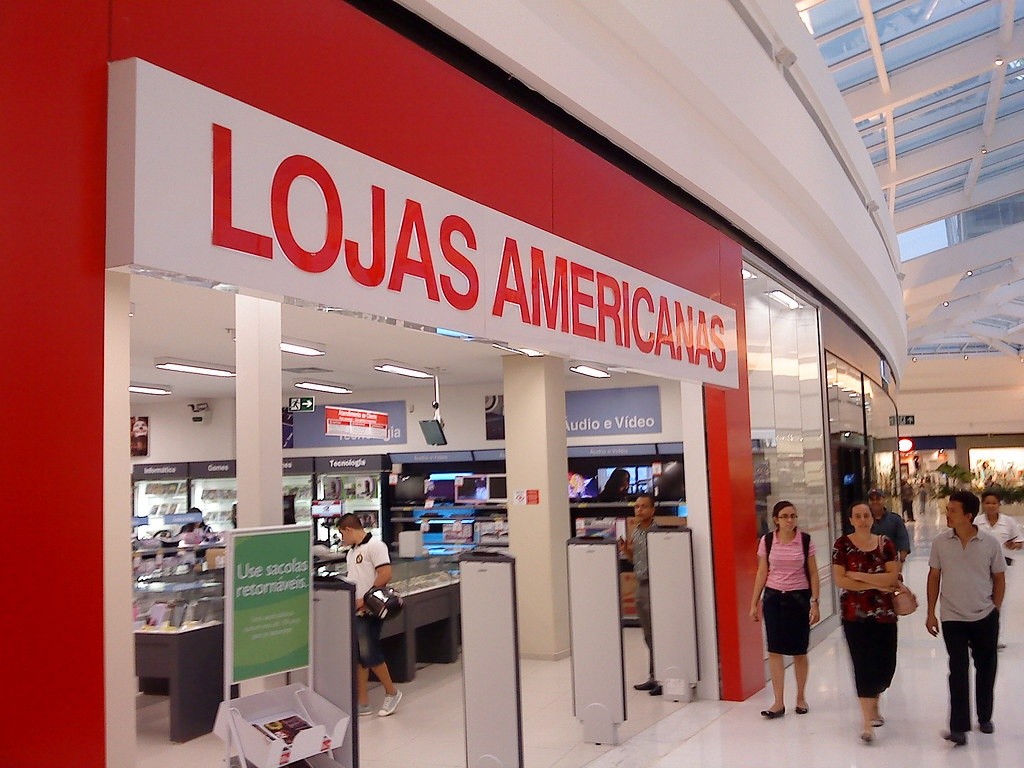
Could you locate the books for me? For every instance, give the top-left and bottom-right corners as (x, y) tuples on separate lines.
(247, 712), (332, 765)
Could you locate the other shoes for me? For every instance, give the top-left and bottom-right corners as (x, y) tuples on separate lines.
(946, 732), (966, 745)
(635, 680), (656, 689)
(650, 686), (662, 696)
(980, 722), (993, 734)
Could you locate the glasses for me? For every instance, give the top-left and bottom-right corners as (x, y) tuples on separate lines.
(778, 515), (798, 519)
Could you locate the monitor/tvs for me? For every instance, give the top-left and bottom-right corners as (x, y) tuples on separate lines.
(396, 473), (508, 506)
(419, 419), (447, 446)
(568, 459), (684, 502)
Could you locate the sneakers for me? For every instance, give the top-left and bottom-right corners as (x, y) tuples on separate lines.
(359, 705), (372, 715)
(378, 689), (403, 716)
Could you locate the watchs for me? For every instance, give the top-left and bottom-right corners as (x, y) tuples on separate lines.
(810, 598), (819, 603)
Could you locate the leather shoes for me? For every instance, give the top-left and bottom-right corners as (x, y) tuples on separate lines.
(761, 707), (785, 718)
(796, 706), (808, 714)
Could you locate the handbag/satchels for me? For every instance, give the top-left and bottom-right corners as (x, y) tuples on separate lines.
(892, 586), (918, 615)
(364, 587), (405, 619)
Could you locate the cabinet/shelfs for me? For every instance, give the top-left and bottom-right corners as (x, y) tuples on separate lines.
(130, 467), (823, 767)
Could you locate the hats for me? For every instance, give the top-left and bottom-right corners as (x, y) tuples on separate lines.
(866, 489), (884, 497)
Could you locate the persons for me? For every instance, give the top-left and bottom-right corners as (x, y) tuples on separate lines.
(336, 513), (404, 716)
(598, 468), (631, 501)
(915, 486), (926, 516)
(926, 491), (1005, 744)
(833, 504), (899, 743)
(864, 489), (912, 585)
(750, 501), (820, 720)
(618, 494), (664, 696)
(983, 462), (991, 488)
(180, 508), (212, 536)
(965, 492), (1024, 651)
(901, 476), (915, 523)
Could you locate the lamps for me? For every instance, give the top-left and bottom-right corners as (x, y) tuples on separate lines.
(897, 272), (904, 281)
(772, 41), (796, 74)
(865, 195), (880, 213)
